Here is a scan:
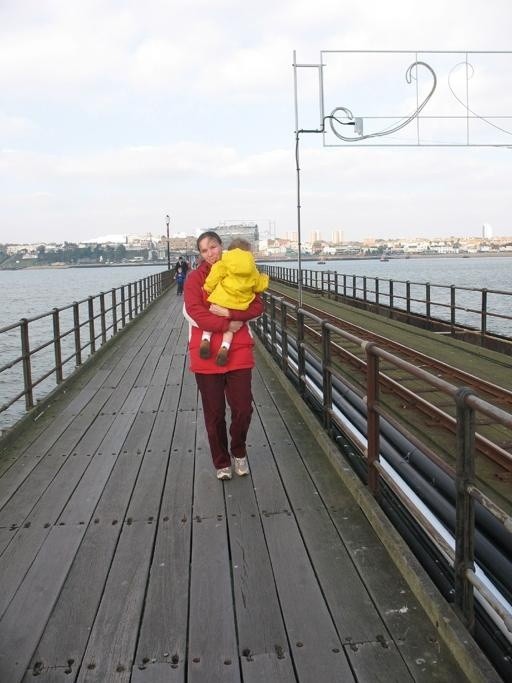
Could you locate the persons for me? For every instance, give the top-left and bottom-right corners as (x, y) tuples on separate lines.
(174, 255), (199, 295)
(182, 231), (265, 482)
(199, 236), (269, 366)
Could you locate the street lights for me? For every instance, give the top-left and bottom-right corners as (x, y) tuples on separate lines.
(184, 239), (187, 256)
(164, 215), (172, 271)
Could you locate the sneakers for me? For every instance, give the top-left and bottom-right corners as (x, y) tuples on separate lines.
(200, 340), (210, 359)
(216, 466), (232, 480)
(216, 346), (228, 366)
(234, 454), (249, 475)
